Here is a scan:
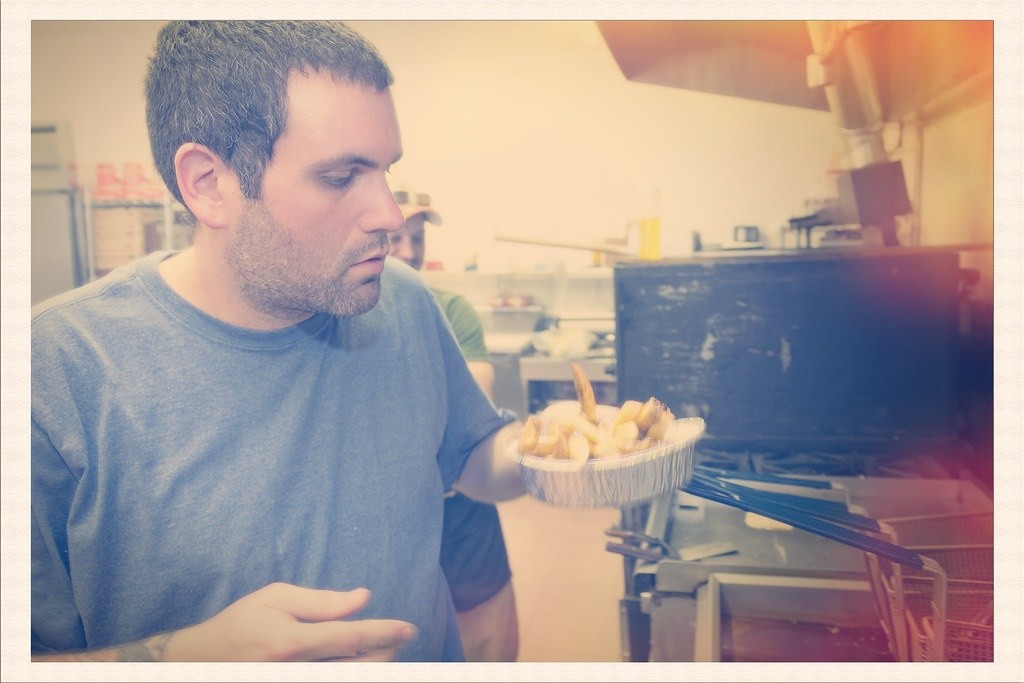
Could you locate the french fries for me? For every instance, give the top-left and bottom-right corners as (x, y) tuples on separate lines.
(521, 397), (675, 466)
(572, 364), (598, 423)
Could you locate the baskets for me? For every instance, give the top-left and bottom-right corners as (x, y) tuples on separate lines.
(506, 416), (707, 506)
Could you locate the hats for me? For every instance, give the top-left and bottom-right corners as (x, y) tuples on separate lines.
(389, 184), (442, 225)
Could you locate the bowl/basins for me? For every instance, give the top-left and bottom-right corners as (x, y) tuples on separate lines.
(504, 414), (706, 510)
(476, 305), (543, 354)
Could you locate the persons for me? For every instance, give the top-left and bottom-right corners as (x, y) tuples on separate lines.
(32, 20), (529, 662)
(385, 201), (520, 662)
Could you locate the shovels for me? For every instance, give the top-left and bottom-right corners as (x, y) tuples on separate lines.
(604, 528), (738, 561)
